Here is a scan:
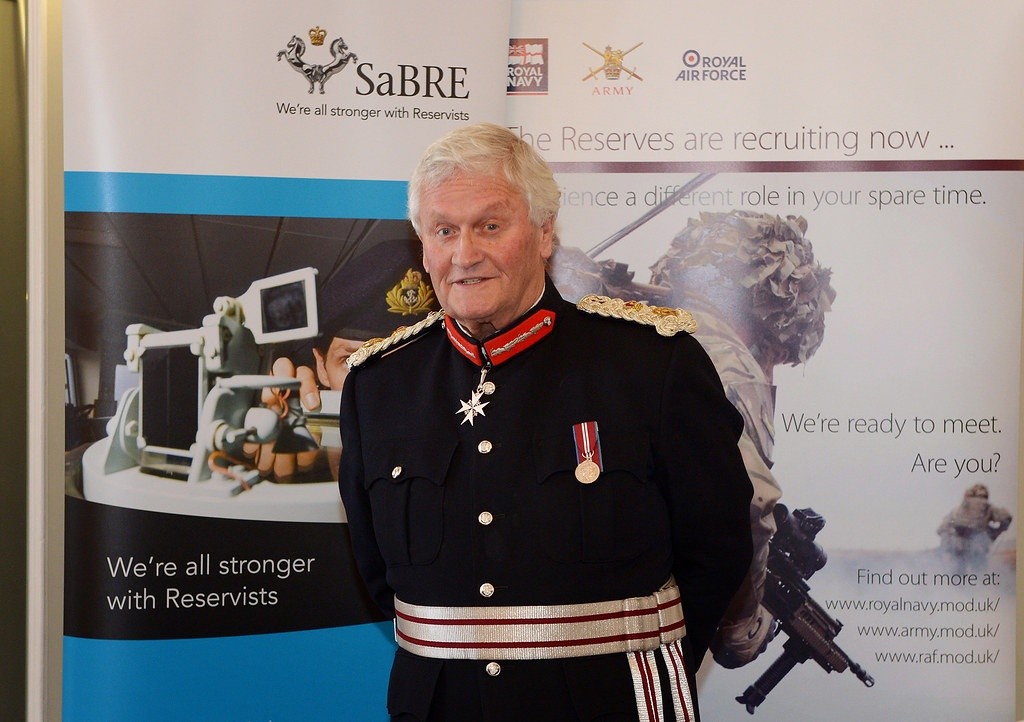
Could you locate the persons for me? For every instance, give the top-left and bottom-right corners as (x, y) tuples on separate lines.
(552, 209), (836, 670)
(260, 238), (444, 411)
(936, 484), (1012, 574)
(336, 124), (763, 722)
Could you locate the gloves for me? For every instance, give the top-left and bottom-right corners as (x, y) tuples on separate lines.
(713, 604), (776, 670)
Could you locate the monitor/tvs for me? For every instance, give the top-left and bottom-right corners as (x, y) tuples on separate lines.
(137, 329), (208, 458)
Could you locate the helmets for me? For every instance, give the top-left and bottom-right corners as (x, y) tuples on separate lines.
(652, 209), (832, 362)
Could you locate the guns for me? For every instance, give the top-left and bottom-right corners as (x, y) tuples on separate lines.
(737, 502), (875, 714)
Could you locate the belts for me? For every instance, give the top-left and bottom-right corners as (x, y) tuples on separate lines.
(392, 579), (687, 661)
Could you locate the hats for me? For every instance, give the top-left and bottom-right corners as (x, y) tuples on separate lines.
(320, 238), (441, 341)
